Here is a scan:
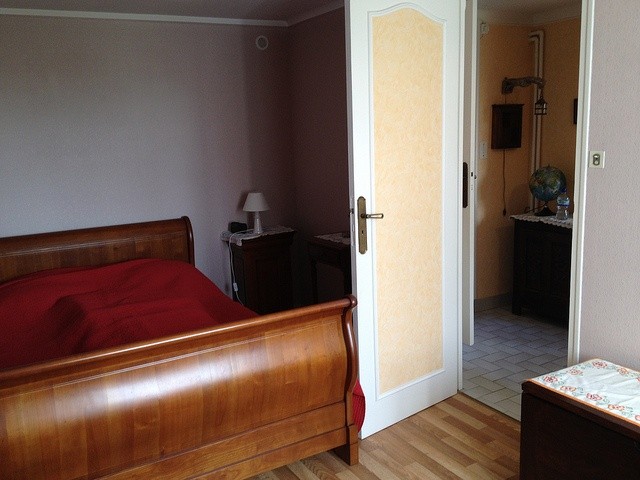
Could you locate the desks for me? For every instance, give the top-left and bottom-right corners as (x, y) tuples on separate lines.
(301, 230), (350, 311)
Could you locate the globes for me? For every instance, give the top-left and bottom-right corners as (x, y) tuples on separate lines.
(529, 164), (566, 216)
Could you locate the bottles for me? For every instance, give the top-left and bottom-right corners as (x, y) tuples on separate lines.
(555, 186), (570, 221)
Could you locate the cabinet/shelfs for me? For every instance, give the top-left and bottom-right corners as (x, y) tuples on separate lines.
(510, 219), (571, 329)
(519, 357), (639, 479)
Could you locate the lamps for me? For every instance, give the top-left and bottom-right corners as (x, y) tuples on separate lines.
(242, 192), (272, 234)
(535, 97), (548, 115)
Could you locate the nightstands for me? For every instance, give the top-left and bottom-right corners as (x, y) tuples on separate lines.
(221, 222), (296, 308)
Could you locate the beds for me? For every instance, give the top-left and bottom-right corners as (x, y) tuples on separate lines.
(1, 215), (365, 480)
